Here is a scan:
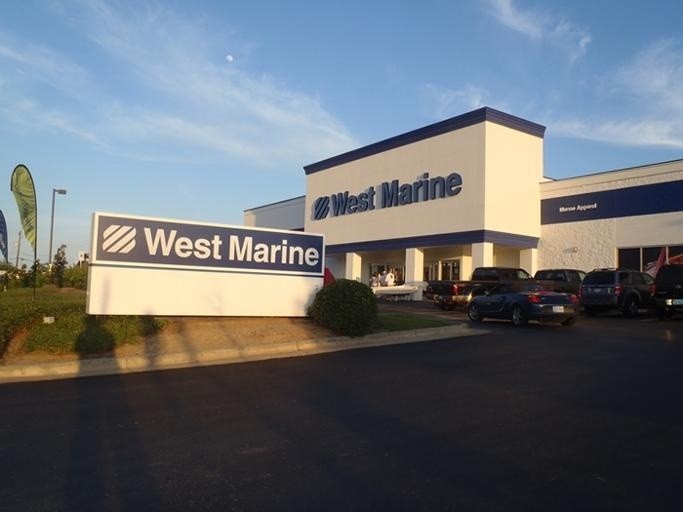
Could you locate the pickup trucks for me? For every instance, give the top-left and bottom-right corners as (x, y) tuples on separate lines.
(423, 267), (534, 310)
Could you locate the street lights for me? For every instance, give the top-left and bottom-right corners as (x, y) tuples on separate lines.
(48, 189), (67, 263)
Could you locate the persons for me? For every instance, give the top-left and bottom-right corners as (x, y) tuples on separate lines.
(367, 265), (401, 306)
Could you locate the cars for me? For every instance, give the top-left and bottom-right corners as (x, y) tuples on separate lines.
(468, 264), (683, 326)
